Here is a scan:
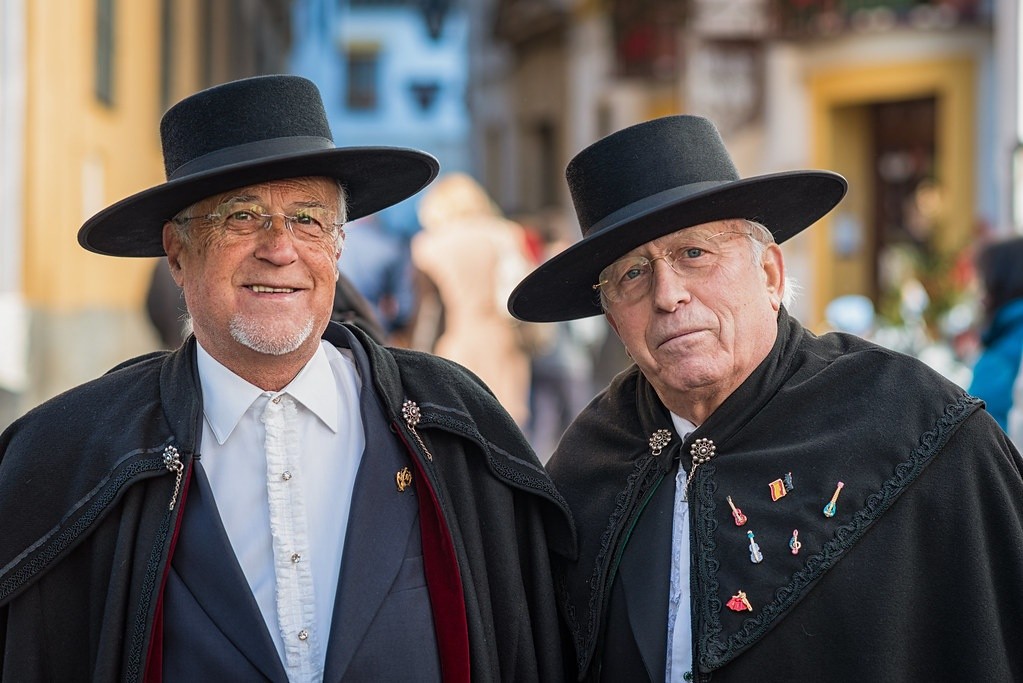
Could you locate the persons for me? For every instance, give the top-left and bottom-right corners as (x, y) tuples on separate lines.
(973, 233), (1022, 452)
(872, 171), (949, 303)
(505, 116), (1021, 683)
(406, 174), (547, 444)
(0, 72), (580, 683)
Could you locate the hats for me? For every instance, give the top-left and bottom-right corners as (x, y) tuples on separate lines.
(506, 114), (850, 323)
(76, 73), (439, 257)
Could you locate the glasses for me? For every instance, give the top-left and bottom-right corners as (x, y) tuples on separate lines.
(172, 202), (347, 244)
(592, 231), (752, 303)
